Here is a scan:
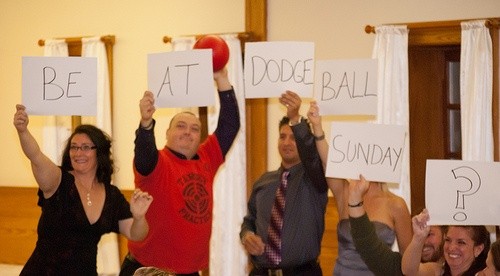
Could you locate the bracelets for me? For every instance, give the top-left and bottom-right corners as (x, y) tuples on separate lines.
(348, 199), (363, 209)
(315, 131), (325, 141)
(142, 121), (153, 128)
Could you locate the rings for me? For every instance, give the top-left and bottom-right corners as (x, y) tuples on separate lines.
(286, 104), (289, 107)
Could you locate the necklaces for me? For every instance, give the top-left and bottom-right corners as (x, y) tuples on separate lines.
(86, 193), (91, 206)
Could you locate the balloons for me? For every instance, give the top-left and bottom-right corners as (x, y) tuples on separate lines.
(193, 35), (230, 72)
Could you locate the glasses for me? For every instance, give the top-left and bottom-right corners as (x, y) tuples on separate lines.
(70, 144), (96, 151)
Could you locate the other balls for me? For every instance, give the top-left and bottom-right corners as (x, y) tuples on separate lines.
(193, 34), (229, 72)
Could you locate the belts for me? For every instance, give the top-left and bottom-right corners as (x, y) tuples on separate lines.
(127, 253), (140, 264)
(260, 261), (318, 276)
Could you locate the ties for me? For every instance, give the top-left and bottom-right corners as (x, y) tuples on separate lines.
(265, 171), (290, 266)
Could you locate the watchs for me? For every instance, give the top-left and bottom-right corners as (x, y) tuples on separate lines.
(288, 115), (303, 126)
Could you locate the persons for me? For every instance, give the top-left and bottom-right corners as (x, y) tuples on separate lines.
(13, 104), (153, 276)
(120, 69), (240, 276)
(401, 208), (500, 276)
(239, 90), (329, 276)
(307, 100), (413, 276)
